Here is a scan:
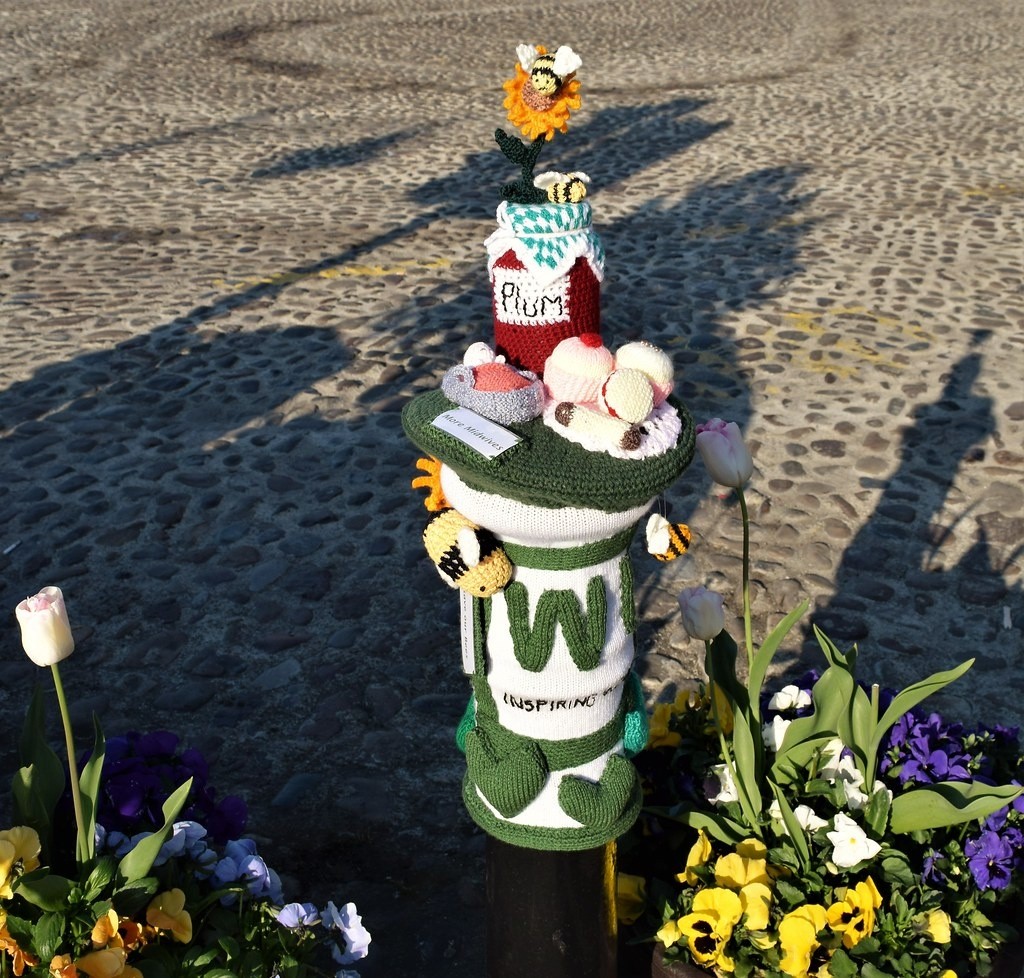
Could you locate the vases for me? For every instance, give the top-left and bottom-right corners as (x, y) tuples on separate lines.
(647, 792), (709, 978)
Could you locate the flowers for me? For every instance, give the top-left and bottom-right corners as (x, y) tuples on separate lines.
(650, 415), (1024, 978)
(0, 586), (371, 978)
